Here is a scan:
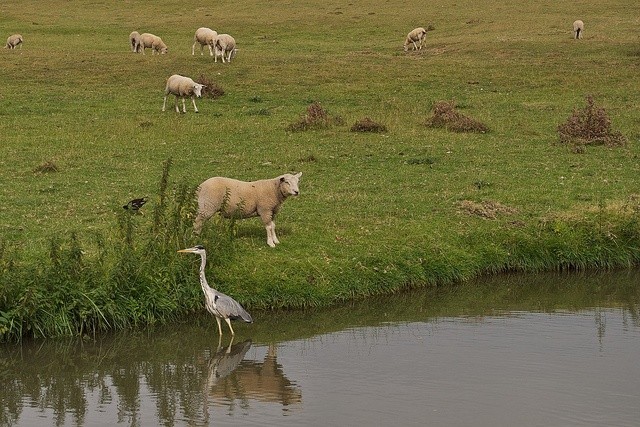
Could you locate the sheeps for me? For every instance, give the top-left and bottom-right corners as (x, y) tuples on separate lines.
(573, 19), (585, 41)
(3, 33), (26, 50)
(128, 31), (140, 54)
(191, 170), (304, 248)
(139, 32), (168, 56)
(161, 74), (208, 115)
(191, 27), (217, 57)
(403, 27), (429, 52)
(213, 32), (242, 65)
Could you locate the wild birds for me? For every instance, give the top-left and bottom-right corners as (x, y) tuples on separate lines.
(123, 195), (151, 218)
(176, 244), (256, 350)
